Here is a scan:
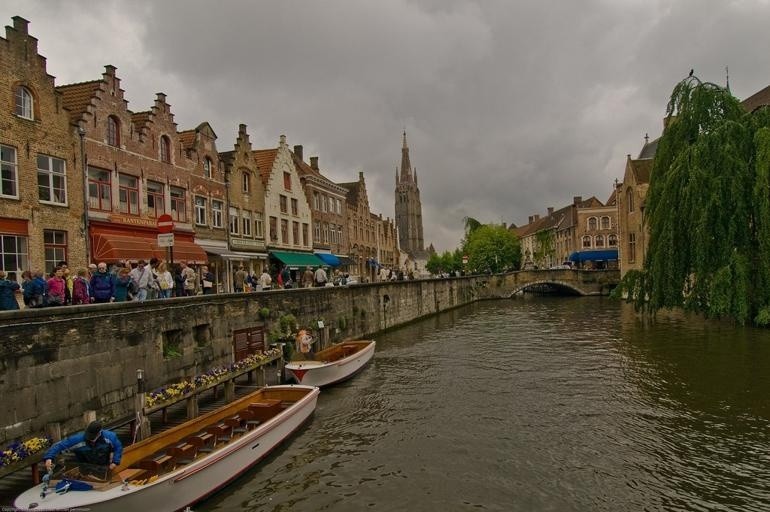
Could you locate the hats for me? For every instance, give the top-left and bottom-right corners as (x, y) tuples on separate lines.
(84, 421), (102, 440)
(150, 257), (162, 269)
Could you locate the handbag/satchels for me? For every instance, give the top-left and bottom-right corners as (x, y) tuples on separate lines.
(27, 295), (43, 307)
(203, 280), (212, 288)
(47, 295), (61, 304)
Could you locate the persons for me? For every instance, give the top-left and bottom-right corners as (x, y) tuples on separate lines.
(45, 419), (125, 485)
(231, 262), (353, 293)
(525, 247), (531, 262)
(378, 263), (421, 282)
(294, 327), (318, 360)
(1, 257), (217, 311)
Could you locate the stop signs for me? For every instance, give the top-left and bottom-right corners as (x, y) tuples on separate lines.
(156, 214), (173, 234)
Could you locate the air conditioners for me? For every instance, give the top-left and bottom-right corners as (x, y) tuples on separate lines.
(6, 270), (25, 289)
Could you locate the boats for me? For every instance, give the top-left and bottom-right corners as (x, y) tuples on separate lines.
(13, 383), (321, 512)
(283, 338), (377, 388)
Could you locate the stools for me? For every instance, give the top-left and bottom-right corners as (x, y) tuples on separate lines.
(322, 343), (360, 361)
(114, 396), (295, 484)
